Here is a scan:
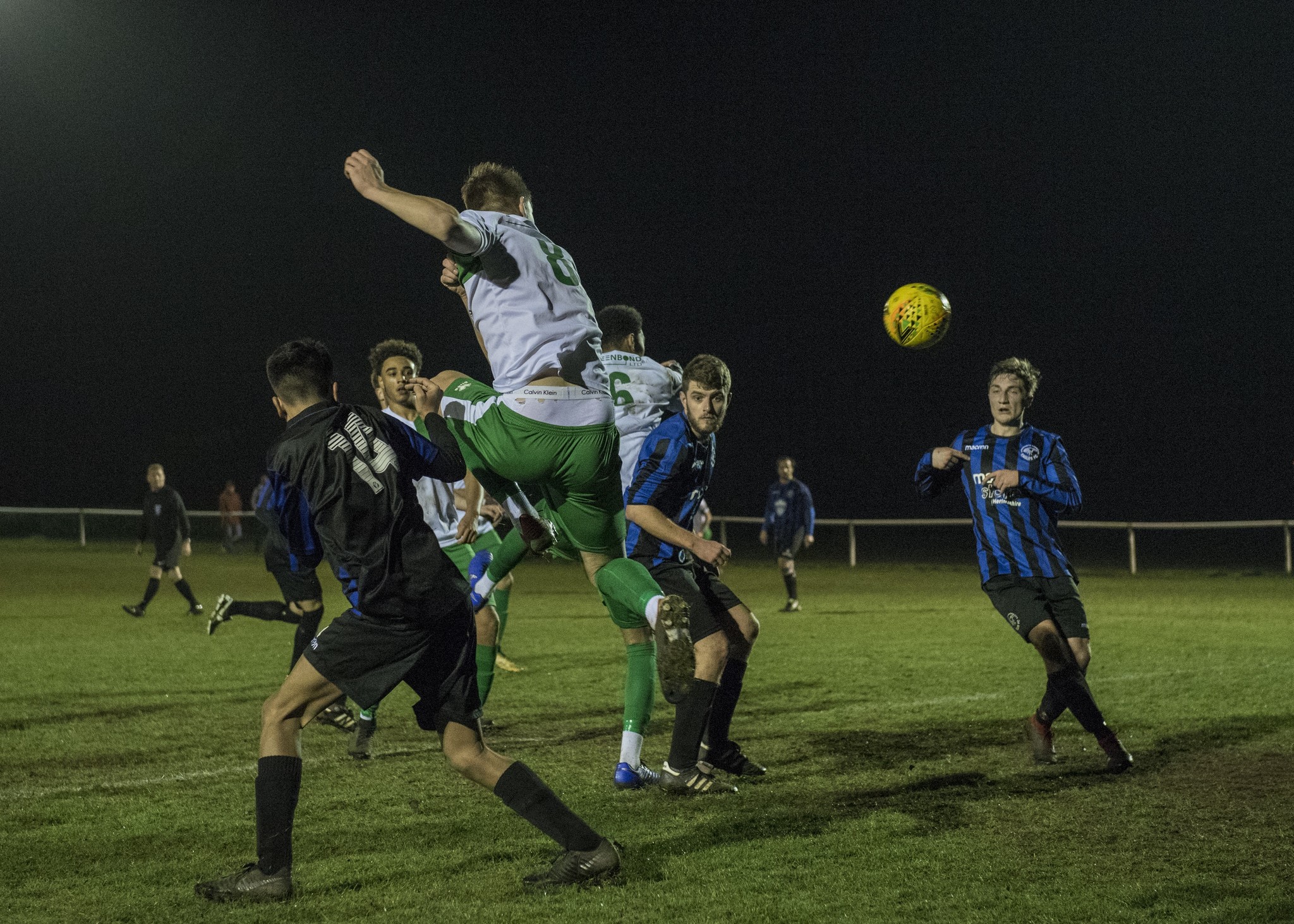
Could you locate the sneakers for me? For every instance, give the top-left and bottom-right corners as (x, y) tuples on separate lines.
(317, 703), (357, 733)
(614, 760), (660, 788)
(194, 864), (293, 902)
(494, 650), (521, 671)
(1030, 708), (1054, 764)
(1100, 735), (1135, 768)
(206, 594), (234, 634)
(660, 762), (738, 794)
(698, 741), (767, 776)
(786, 598), (800, 611)
(519, 515), (558, 563)
(524, 837), (621, 888)
(349, 720), (377, 759)
(468, 550), (493, 614)
(186, 602), (205, 615)
(656, 595), (697, 704)
(122, 605), (146, 618)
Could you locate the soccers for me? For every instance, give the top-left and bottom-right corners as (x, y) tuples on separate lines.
(883, 282), (953, 347)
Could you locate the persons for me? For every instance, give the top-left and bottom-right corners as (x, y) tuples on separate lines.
(621, 355), (767, 794)
(251, 473), (273, 555)
(693, 498), (720, 577)
(350, 337), (500, 757)
(345, 149), (694, 704)
(759, 456), (814, 611)
(194, 341), (625, 904)
(220, 480), (242, 553)
(370, 378), (529, 673)
(471, 303), (685, 792)
(915, 356), (1134, 767)
(121, 464), (204, 618)
(207, 480), (357, 731)
